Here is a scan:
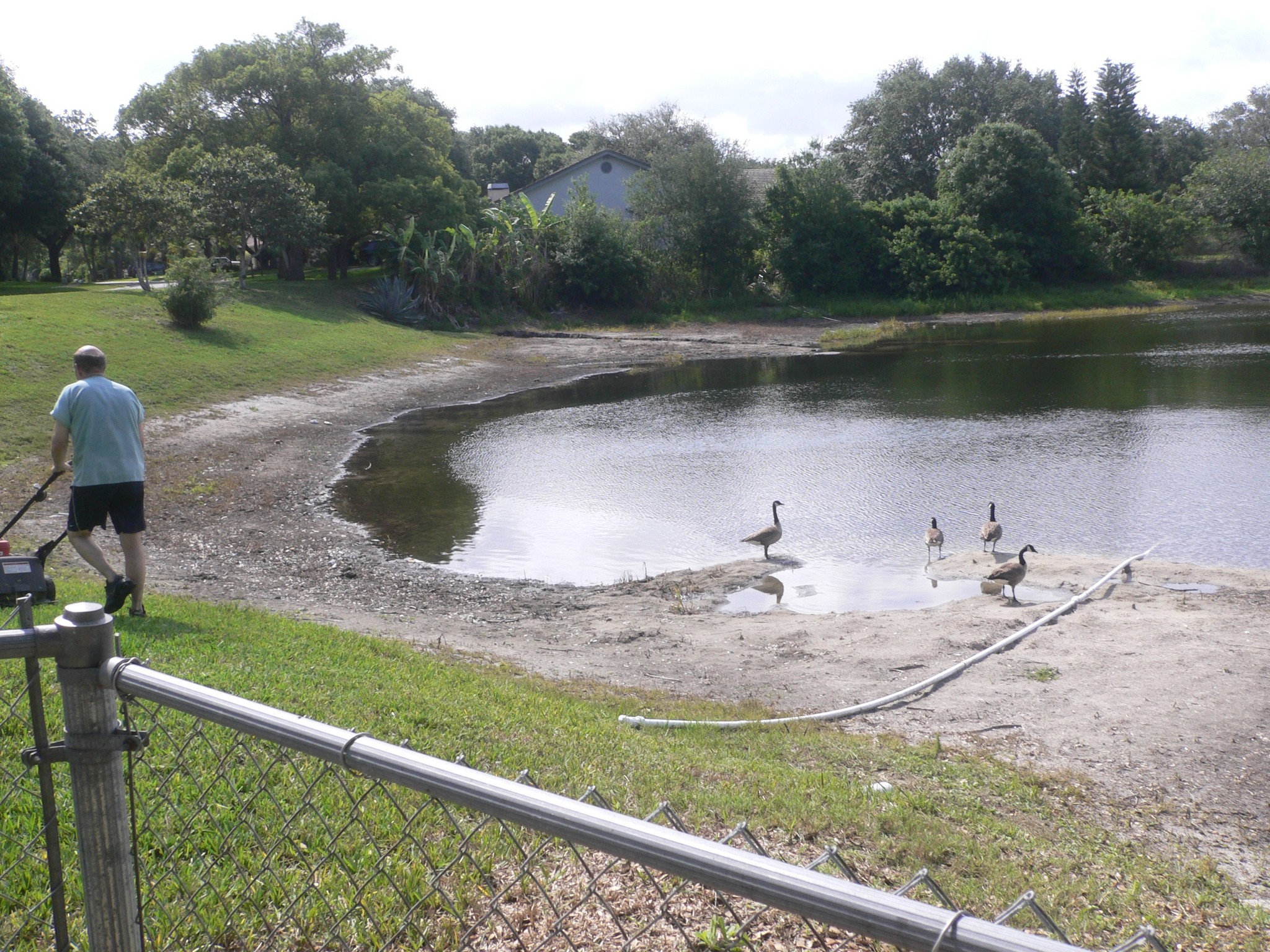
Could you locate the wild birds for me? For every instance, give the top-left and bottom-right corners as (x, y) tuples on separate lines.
(740, 500), (784, 559)
(980, 501), (1002, 553)
(983, 544), (1039, 605)
(980, 581), (1002, 597)
(751, 575), (784, 604)
(924, 551), (942, 589)
(924, 517), (944, 552)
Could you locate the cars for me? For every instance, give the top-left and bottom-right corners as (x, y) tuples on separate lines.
(208, 257), (241, 273)
(128, 261), (169, 278)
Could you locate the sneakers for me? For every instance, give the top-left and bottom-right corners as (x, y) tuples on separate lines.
(126, 602), (147, 619)
(102, 571), (136, 614)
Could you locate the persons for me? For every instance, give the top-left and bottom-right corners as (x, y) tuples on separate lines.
(49, 346), (147, 618)
